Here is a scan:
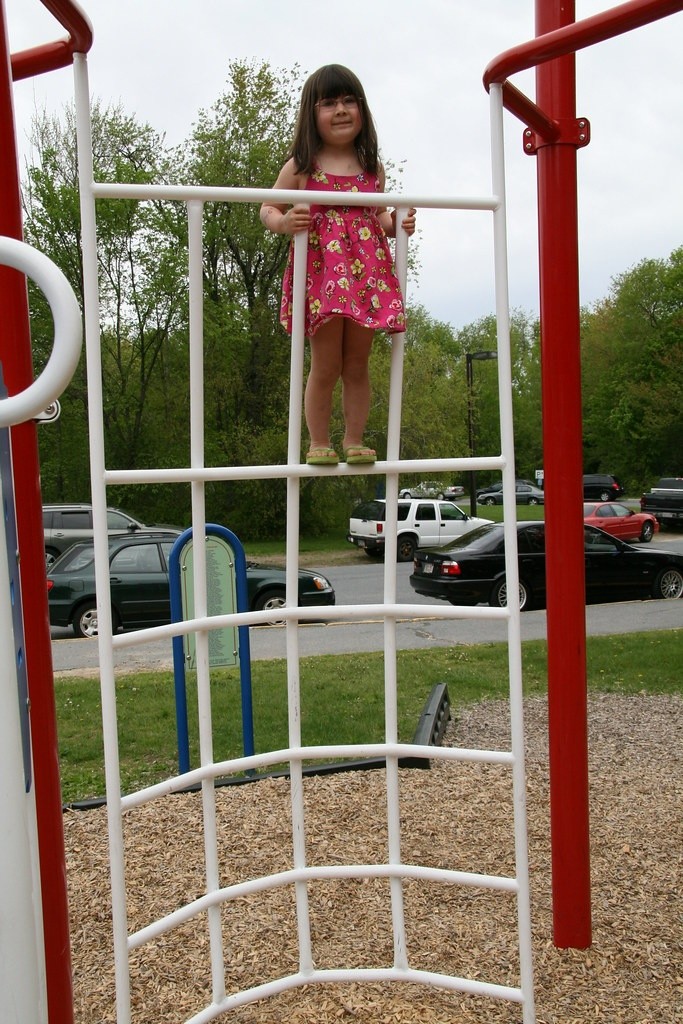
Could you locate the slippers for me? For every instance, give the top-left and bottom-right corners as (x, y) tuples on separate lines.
(343, 446), (377, 463)
(306, 448), (339, 464)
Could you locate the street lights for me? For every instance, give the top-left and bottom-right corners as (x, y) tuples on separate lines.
(464, 350), (498, 518)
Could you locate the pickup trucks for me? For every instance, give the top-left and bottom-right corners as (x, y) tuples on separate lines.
(641, 477), (683, 532)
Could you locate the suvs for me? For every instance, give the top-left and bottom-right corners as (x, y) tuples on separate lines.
(40, 501), (187, 571)
(346, 497), (495, 562)
(582, 473), (625, 501)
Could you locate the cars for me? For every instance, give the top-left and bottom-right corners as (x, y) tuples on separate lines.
(474, 479), (545, 507)
(48, 531), (337, 638)
(581, 501), (660, 543)
(409, 519), (683, 612)
(399, 480), (465, 500)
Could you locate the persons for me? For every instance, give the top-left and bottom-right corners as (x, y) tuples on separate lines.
(260, 64), (417, 465)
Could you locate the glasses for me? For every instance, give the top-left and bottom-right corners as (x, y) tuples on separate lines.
(313, 95), (363, 113)
